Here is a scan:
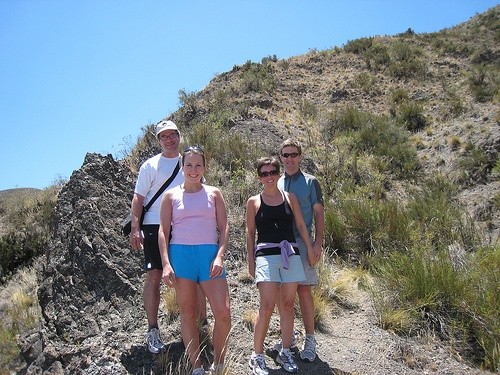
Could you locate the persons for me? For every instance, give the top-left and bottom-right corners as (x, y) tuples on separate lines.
(158, 145), (233, 375)
(274, 139), (324, 363)
(129, 120), (211, 353)
(245, 156), (315, 375)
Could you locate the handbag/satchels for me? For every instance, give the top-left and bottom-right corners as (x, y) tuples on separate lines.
(120, 206), (145, 236)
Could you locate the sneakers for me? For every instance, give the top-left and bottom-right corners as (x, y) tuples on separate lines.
(249, 352), (270, 375)
(146, 328), (166, 353)
(300, 336), (316, 363)
(276, 351), (299, 373)
(274, 336), (297, 352)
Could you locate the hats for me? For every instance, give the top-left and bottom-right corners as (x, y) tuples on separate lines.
(156, 120), (179, 139)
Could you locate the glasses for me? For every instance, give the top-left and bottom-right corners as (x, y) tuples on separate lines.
(260, 170), (277, 178)
(282, 153), (299, 158)
(160, 133), (178, 140)
